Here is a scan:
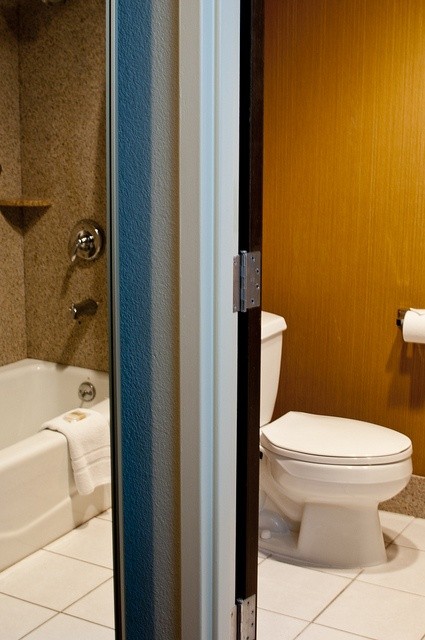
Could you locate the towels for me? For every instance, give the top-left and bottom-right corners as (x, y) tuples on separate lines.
(37, 406), (111, 496)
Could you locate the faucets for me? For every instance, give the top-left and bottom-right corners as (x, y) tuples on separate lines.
(69, 299), (98, 320)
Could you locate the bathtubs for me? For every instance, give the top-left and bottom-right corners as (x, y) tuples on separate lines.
(0, 356), (113, 577)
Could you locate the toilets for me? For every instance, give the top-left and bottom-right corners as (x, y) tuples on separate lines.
(258, 306), (414, 571)
